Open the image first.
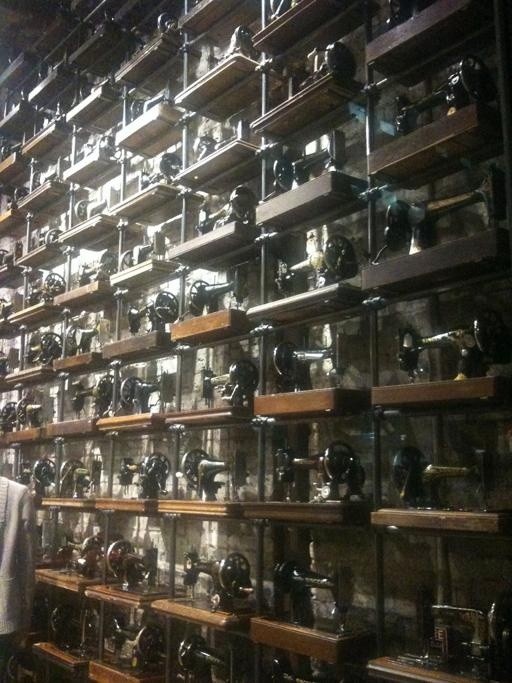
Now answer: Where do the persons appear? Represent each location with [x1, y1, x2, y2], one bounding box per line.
[0, 474, 38, 683]
